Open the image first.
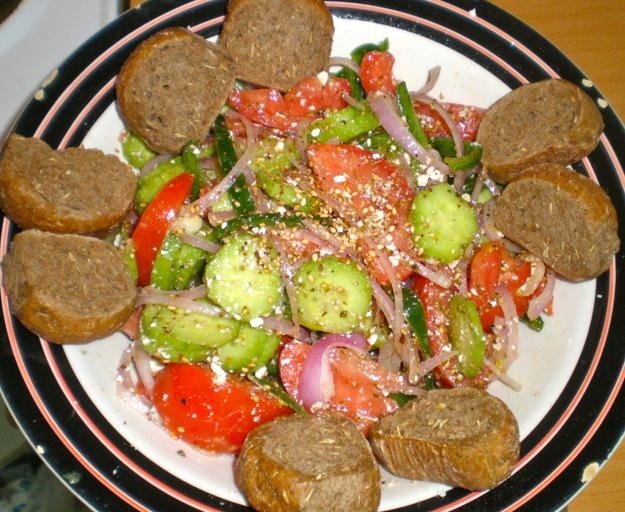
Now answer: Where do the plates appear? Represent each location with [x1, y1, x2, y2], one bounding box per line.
[1, 1, 625, 511]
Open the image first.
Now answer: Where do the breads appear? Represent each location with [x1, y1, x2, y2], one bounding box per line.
[231, 413, 382, 511]
[0, 133, 138, 234]
[474, 78, 603, 185]
[1, 229, 137, 345]
[493, 163, 620, 283]
[369, 389, 523, 492]
[111, 28, 240, 157]
[221, 3, 334, 87]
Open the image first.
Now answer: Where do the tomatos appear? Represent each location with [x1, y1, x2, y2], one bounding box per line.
[123, 51, 551, 455]
